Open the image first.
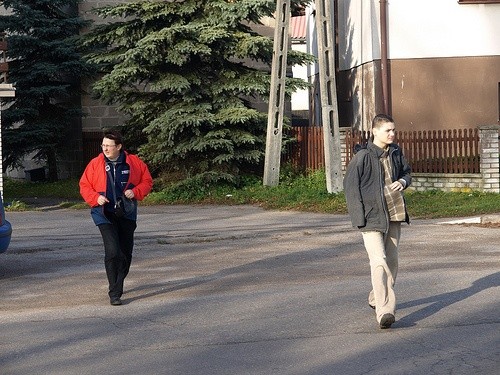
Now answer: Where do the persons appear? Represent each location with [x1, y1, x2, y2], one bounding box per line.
[80, 127, 152, 306]
[343, 113, 413, 329]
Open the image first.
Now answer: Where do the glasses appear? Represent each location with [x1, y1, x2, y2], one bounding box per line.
[100, 145, 115, 148]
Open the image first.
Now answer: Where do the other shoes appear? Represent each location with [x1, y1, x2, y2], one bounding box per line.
[111, 297, 122, 304]
[380, 313, 395, 329]
[369, 304, 375, 309]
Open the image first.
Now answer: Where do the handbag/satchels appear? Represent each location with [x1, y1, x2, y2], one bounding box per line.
[106, 163, 134, 217]
